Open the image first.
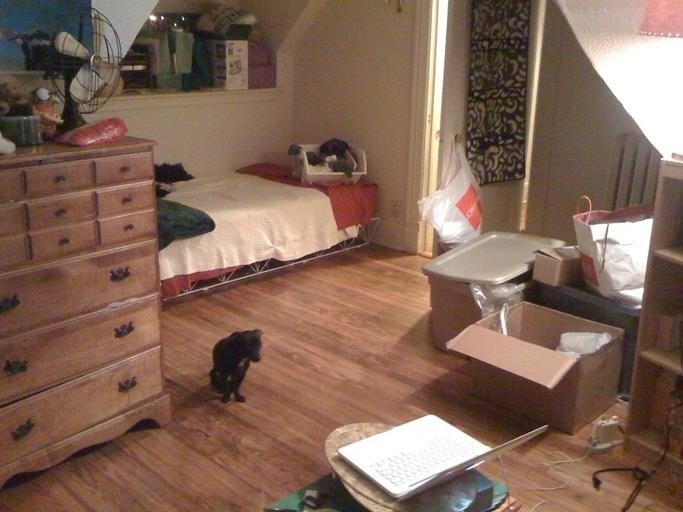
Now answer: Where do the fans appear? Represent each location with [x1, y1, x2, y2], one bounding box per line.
[40, 2, 124, 136]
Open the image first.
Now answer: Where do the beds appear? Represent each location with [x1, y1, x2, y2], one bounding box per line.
[152, 158, 383, 301]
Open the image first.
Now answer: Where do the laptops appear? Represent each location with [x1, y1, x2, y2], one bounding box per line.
[336, 414, 549, 501]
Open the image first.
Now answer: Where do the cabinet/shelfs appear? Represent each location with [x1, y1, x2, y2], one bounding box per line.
[0, 125, 174, 486]
[621, 149, 682, 471]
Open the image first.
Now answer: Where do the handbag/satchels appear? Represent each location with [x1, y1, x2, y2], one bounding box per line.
[572, 201, 654, 318]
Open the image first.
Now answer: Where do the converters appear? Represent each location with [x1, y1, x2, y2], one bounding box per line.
[598, 418, 618, 443]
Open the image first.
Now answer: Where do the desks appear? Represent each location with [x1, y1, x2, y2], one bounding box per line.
[259, 463, 525, 511]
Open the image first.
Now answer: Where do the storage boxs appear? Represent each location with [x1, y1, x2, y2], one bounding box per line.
[204, 37, 250, 93]
[290, 139, 367, 185]
[420, 229, 571, 357]
[529, 243, 581, 289]
[444, 298, 627, 437]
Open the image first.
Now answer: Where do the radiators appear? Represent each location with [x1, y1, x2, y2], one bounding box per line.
[605, 130, 662, 216]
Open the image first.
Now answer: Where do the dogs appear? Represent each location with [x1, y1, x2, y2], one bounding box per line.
[207, 328, 264, 402]
